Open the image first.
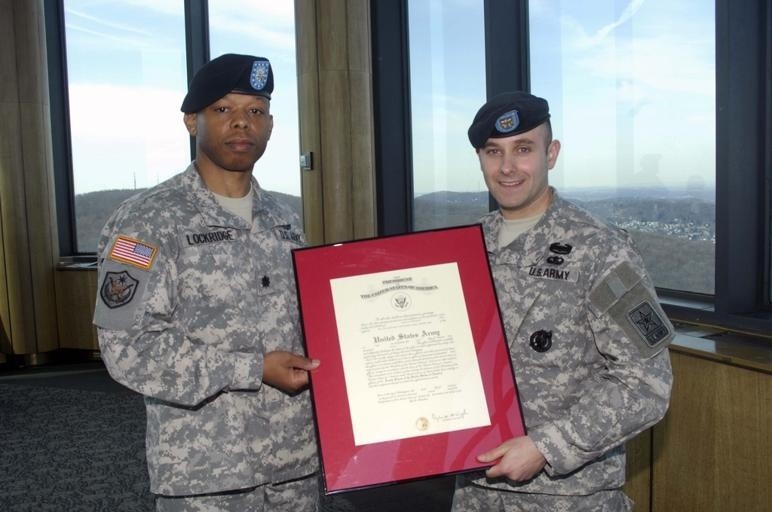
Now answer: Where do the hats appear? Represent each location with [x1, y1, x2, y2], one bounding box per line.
[181, 53, 274, 115]
[467, 92, 552, 148]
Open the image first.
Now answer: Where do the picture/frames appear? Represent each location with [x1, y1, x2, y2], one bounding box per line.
[290, 221, 528, 497]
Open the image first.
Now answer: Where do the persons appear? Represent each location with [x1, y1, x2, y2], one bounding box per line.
[92, 53, 321, 512]
[450, 92, 676, 512]
